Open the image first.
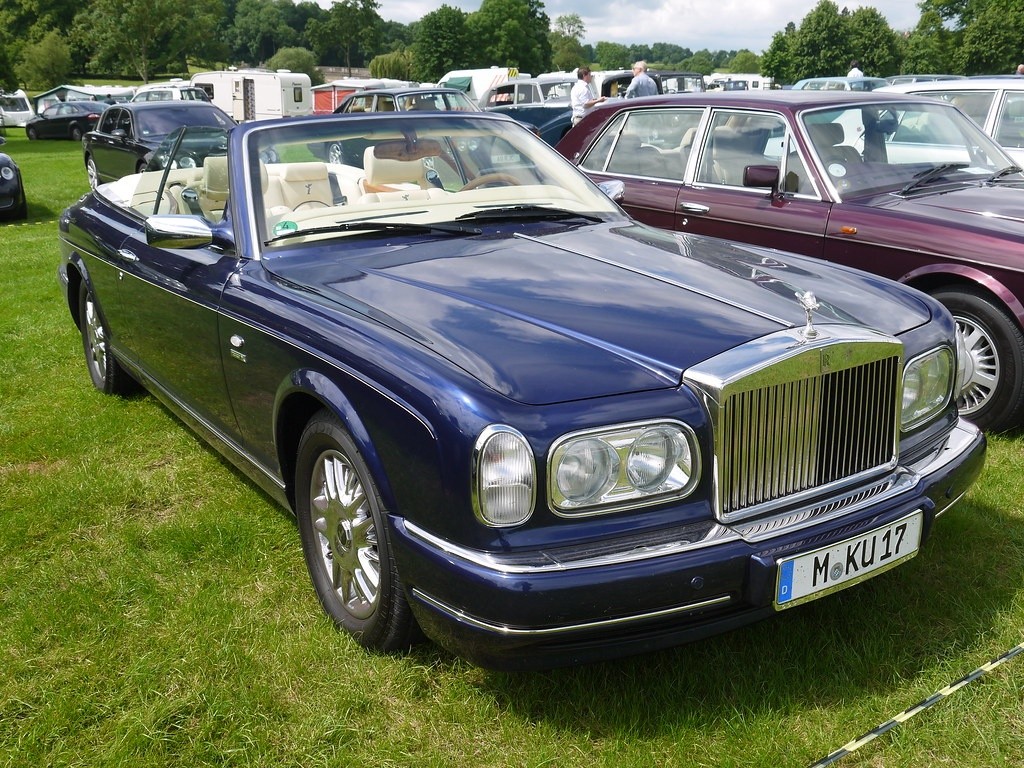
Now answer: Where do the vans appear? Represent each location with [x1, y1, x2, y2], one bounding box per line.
[191, 63, 1024, 172]
[128, 87, 211, 103]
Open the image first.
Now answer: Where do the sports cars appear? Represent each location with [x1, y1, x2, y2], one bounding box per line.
[58, 112, 987, 672]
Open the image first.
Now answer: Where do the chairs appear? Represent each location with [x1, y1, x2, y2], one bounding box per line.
[950, 95, 1023, 146]
[349, 99, 440, 115]
[594, 123, 863, 198]
[168, 147, 453, 225]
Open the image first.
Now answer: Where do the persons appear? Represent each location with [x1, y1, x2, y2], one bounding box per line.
[844, 61, 864, 90]
[1015, 64, 1024, 75]
[624, 61, 658, 144]
[570, 67, 607, 128]
[104, 94, 116, 105]
[90, 96, 95, 101]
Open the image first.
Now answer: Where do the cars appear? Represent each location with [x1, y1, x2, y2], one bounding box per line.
[84, 101, 238, 191]
[26, 101, 111, 141]
[554, 90, 1024, 433]
